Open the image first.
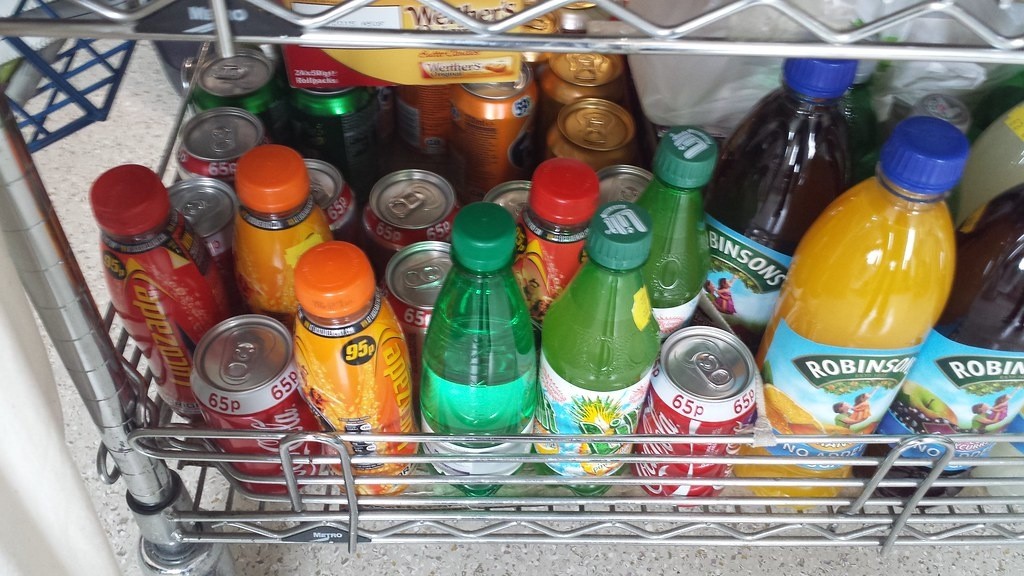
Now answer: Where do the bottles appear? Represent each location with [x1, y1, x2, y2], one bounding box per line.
[419, 201, 537, 503]
[699, 40, 883, 365]
[629, 125, 718, 345]
[534, 202, 660, 497]
[513, 157, 613, 354]
[288, 239, 421, 503]
[727, 92, 1023, 517]
[89, 164, 235, 440]
[229, 142, 337, 327]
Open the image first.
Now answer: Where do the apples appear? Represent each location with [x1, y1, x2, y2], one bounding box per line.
[901, 382, 957, 425]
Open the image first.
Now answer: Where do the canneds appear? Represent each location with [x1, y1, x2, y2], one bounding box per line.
[164, 0, 761, 498]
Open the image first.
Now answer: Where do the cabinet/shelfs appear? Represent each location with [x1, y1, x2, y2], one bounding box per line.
[0, 0, 1024, 576]
